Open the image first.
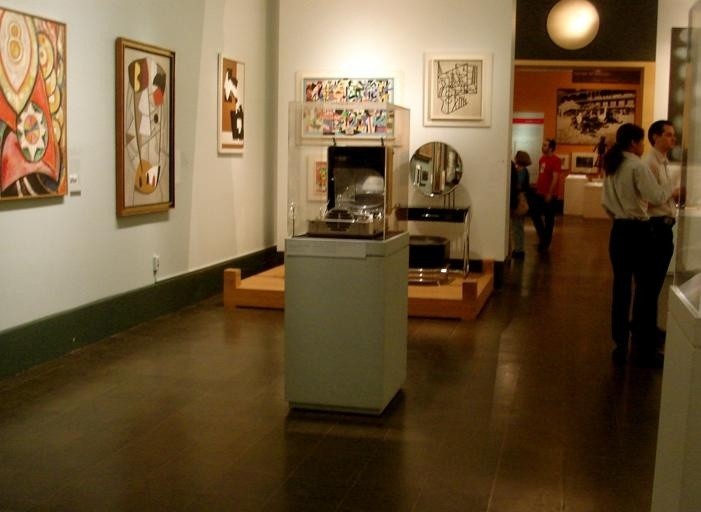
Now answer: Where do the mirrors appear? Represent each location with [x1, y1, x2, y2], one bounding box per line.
[408, 141, 463, 208]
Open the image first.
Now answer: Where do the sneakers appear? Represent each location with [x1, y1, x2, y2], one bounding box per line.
[612, 328, 665, 366]
[536, 242, 551, 252]
[512, 249, 525, 257]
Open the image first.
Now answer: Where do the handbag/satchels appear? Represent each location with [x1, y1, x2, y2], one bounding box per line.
[514, 192, 530, 216]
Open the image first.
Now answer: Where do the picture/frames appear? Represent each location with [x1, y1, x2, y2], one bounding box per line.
[116, 37, 176, 217]
[423, 49, 493, 128]
[0, 6, 69, 200]
[571, 151, 598, 174]
[217, 51, 246, 154]
[296, 71, 395, 139]
[307, 151, 329, 203]
[410, 142, 462, 198]
[572, 68, 641, 84]
[556, 155, 569, 170]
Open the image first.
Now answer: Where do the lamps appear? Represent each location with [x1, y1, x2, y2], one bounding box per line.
[546, 0, 600, 51]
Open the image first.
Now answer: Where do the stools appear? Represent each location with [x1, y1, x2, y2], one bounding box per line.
[408, 235, 451, 286]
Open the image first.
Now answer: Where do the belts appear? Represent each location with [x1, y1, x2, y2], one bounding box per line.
[654, 215, 675, 226]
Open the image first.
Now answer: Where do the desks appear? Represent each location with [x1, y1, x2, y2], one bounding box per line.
[408, 205, 471, 279]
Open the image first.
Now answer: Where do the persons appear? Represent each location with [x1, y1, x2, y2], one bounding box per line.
[512, 150, 533, 258]
[638, 120, 682, 345]
[535, 139, 562, 251]
[600, 124, 678, 370]
